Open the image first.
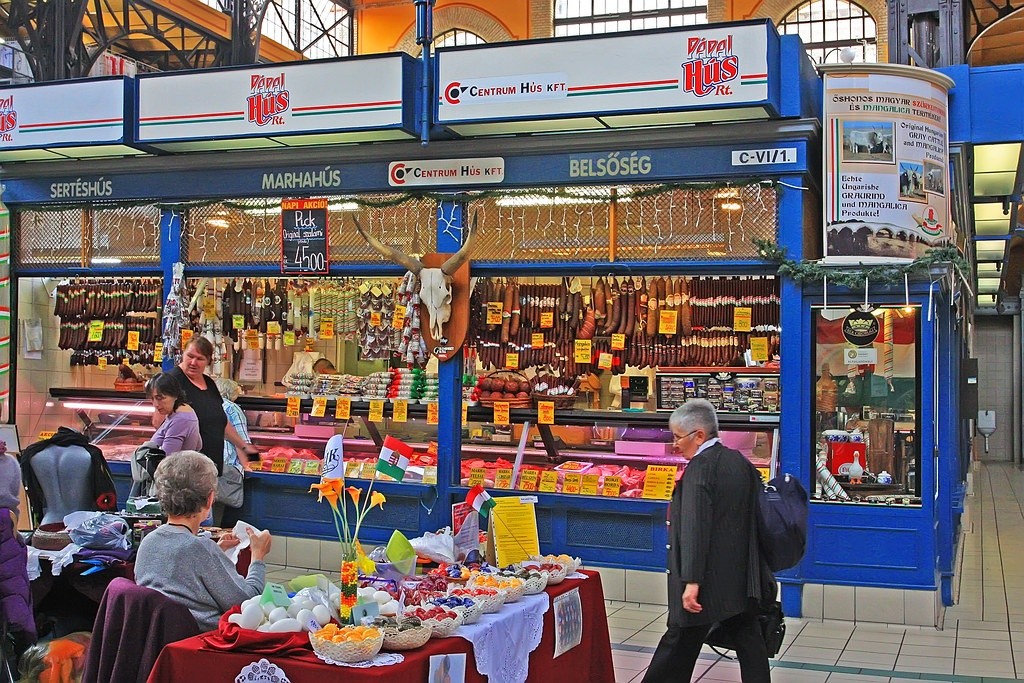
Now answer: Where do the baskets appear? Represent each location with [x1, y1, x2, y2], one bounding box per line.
[361, 614, 432, 650]
[421, 555, 581, 636]
[530, 391, 577, 409]
[475, 369, 533, 408]
[309, 624, 385, 662]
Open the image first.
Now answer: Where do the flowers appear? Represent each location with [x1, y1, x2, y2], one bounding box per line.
[308, 477, 386, 561]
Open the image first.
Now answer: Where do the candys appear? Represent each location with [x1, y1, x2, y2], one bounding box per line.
[314, 623, 380, 654]
[379, 562, 521, 621]
[524, 554, 572, 578]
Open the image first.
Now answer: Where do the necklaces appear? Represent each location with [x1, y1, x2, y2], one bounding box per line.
[167, 523, 192, 534]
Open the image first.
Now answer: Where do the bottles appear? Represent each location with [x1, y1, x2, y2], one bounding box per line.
[849, 451, 863, 479]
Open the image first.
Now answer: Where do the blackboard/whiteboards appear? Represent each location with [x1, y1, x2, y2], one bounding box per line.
[281, 208, 328, 274]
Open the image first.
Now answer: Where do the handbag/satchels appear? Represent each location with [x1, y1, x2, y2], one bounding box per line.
[758, 474, 807, 569]
[703, 600, 786, 658]
[216, 466, 243, 508]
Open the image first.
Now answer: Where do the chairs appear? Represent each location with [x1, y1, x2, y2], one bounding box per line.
[79, 576, 201, 683]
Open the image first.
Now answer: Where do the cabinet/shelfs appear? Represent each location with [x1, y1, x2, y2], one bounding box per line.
[654, 367, 780, 415]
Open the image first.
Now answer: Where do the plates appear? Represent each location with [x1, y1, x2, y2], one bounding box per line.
[553, 461, 593, 473]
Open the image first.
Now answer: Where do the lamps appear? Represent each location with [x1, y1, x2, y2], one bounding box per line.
[807, 46, 856, 64]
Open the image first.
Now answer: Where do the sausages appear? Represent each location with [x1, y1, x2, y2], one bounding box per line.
[54, 280, 163, 384]
[531, 374, 581, 397]
[468, 277, 780, 379]
[189, 272, 430, 377]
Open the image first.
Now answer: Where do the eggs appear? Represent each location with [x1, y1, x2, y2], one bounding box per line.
[228, 594, 330, 632]
[331, 587, 399, 614]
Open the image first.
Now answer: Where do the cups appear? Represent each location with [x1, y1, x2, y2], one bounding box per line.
[849, 432, 861, 443]
[877, 471, 891, 484]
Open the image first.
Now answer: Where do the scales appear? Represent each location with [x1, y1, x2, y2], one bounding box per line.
[615, 376, 676, 457]
[531, 435, 566, 450]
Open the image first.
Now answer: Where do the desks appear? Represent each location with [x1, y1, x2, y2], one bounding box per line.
[21, 518, 251, 637]
[147, 547, 616, 683]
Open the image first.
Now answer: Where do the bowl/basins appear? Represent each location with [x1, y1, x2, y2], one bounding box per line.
[359, 555, 418, 581]
[595, 422, 628, 440]
[822, 432, 848, 443]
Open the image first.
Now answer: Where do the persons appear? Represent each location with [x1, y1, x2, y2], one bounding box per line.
[640, 398, 770, 683]
[312, 359, 343, 375]
[170, 335, 258, 527]
[145, 371, 202, 460]
[213, 378, 252, 528]
[133, 452, 273, 633]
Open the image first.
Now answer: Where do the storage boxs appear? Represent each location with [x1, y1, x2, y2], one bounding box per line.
[295, 424, 360, 439]
[358, 553, 419, 592]
[615, 441, 675, 456]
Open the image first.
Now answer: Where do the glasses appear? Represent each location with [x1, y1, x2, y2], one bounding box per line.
[673, 430, 697, 441]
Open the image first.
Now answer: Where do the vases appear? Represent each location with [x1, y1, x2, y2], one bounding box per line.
[338, 553, 359, 626]
